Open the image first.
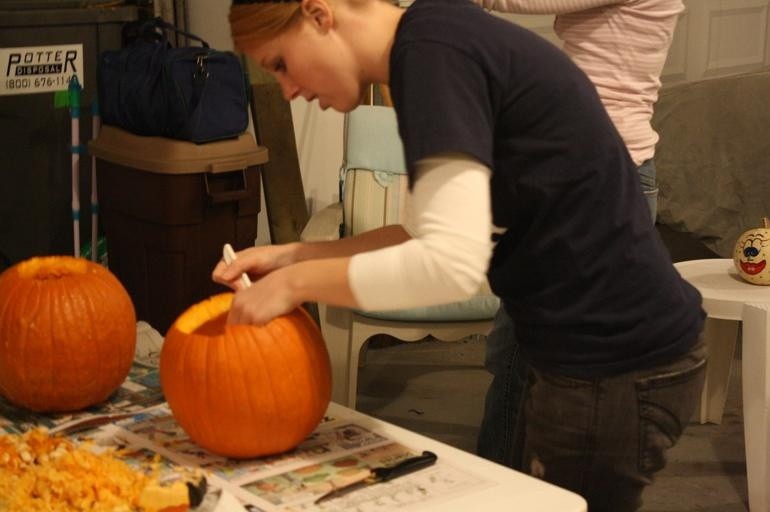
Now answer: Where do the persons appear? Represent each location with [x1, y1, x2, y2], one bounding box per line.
[467, 0, 686, 473]
[210, 0, 711, 511]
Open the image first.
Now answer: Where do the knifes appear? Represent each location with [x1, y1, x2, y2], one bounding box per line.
[311, 451, 438, 505]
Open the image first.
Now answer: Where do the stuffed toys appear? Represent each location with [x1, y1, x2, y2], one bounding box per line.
[733, 227, 770, 286]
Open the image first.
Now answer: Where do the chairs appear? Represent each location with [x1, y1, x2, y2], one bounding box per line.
[300, 105, 501, 410]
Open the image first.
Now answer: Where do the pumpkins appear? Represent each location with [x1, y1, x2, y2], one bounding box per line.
[160, 290, 333, 457]
[0, 255, 136, 413]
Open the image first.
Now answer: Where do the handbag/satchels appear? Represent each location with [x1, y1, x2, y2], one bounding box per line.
[96, 16, 249, 144]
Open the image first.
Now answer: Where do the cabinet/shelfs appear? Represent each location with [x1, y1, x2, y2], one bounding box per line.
[0, 0, 140, 272]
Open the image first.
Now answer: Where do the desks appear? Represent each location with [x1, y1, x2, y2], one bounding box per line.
[672, 257, 770, 512]
[0, 355, 588, 512]
